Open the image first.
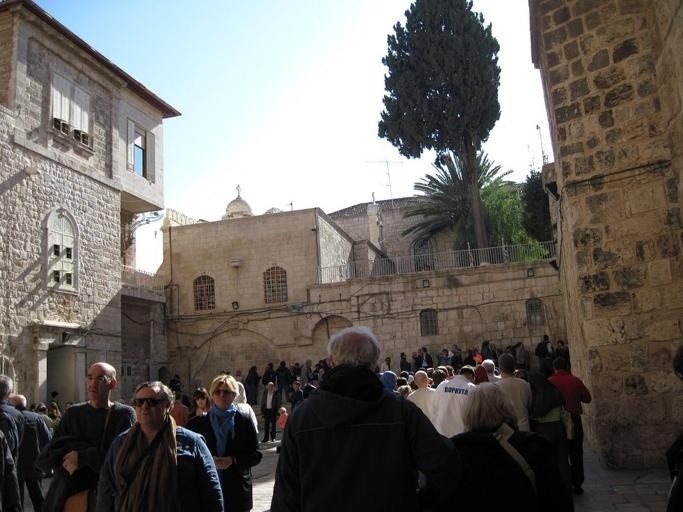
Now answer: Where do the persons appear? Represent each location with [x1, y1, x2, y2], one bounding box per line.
[664, 345, 683, 512]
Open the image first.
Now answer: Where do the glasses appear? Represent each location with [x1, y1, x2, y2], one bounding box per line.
[214, 390, 230, 395]
[87, 377, 104, 381]
[133, 399, 169, 407]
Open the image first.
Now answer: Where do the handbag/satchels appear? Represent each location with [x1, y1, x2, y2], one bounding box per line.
[63, 489, 88, 512]
[561, 405, 575, 440]
